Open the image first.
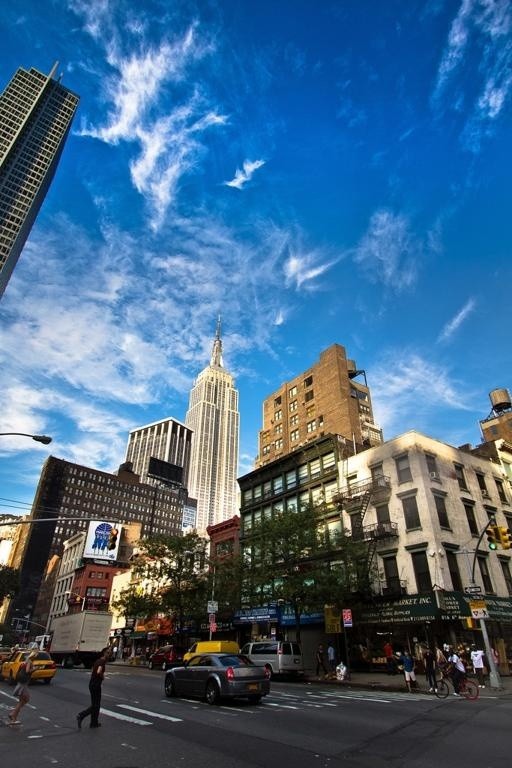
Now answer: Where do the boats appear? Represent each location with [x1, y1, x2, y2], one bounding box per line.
[485, 527, 498, 550]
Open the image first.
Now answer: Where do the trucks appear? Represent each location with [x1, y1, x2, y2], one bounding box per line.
[46, 610, 114, 669]
[30, 635, 51, 651]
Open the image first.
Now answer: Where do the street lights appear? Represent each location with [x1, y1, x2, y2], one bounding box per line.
[184, 549, 216, 641]
[65, 589, 86, 611]
[0, 431, 53, 445]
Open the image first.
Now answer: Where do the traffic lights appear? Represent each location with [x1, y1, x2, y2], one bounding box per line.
[108, 528, 119, 550]
[11, 619, 15, 626]
[498, 526, 512, 550]
[461, 619, 471, 628]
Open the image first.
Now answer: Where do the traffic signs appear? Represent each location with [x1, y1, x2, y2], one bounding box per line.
[463, 585, 483, 594]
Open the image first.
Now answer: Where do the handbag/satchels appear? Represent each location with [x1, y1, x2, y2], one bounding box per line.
[15, 668, 29, 684]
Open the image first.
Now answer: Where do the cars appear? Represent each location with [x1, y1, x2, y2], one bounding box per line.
[146, 638, 305, 707]
[0, 645, 57, 684]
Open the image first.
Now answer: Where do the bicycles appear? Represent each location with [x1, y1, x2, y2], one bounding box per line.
[430, 664, 482, 700]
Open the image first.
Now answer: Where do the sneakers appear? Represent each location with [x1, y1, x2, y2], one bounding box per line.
[7, 714, 24, 726]
[75, 715, 102, 730]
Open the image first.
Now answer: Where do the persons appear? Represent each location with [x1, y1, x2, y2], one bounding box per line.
[77, 647, 110, 731]
[113, 646, 151, 662]
[316, 642, 499, 696]
[8, 648, 40, 725]
